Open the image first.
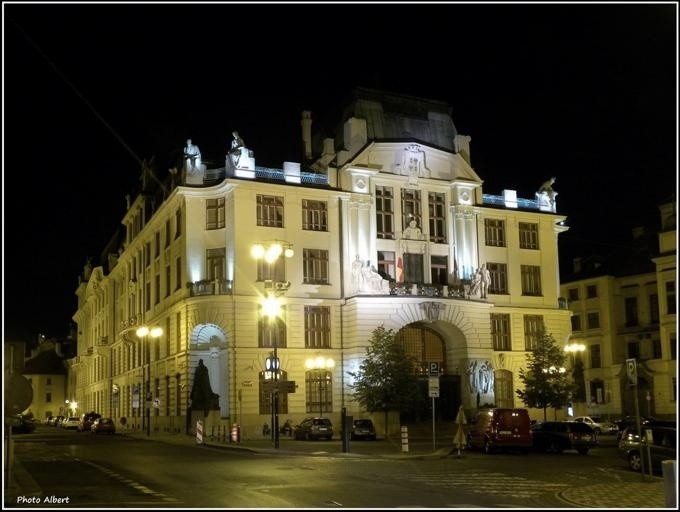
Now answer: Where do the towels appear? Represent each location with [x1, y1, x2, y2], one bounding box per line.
[662, 460, 676, 507]
[229, 426, 240, 441]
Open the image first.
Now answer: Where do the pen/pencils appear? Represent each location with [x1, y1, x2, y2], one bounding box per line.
[468, 408, 533, 454]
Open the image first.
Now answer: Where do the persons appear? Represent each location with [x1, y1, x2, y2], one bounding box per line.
[282, 418, 294, 438]
[190, 358, 219, 413]
[470, 268, 482, 296]
[464, 360, 495, 395]
[262, 422, 271, 437]
[477, 262, 491, 300]
[360, 259, 381, 291]
[183, 138, 202, 177]
[82, 260, 93, 281]
[402, 220, 422, 240]
[54, 416, 62, 432]
[227, 130, 255, 169]
[539, 176, 558, 208]
[351, 253, 364, 293]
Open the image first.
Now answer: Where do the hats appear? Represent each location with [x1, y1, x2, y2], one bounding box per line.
[45, 413, 116, 434]
[17, 415, 35, 432]
[294, 416, 334, 441]
[531, 415, 676, 471]
[351, 419, 376, 440]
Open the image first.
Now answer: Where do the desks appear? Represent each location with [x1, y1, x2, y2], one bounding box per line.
[540, 365, 565, 421]
[263, 351, 282, 443]
[255, 237, 295, 449]
[136, 324, 162, 437]
[563, 342, 586, 415]
